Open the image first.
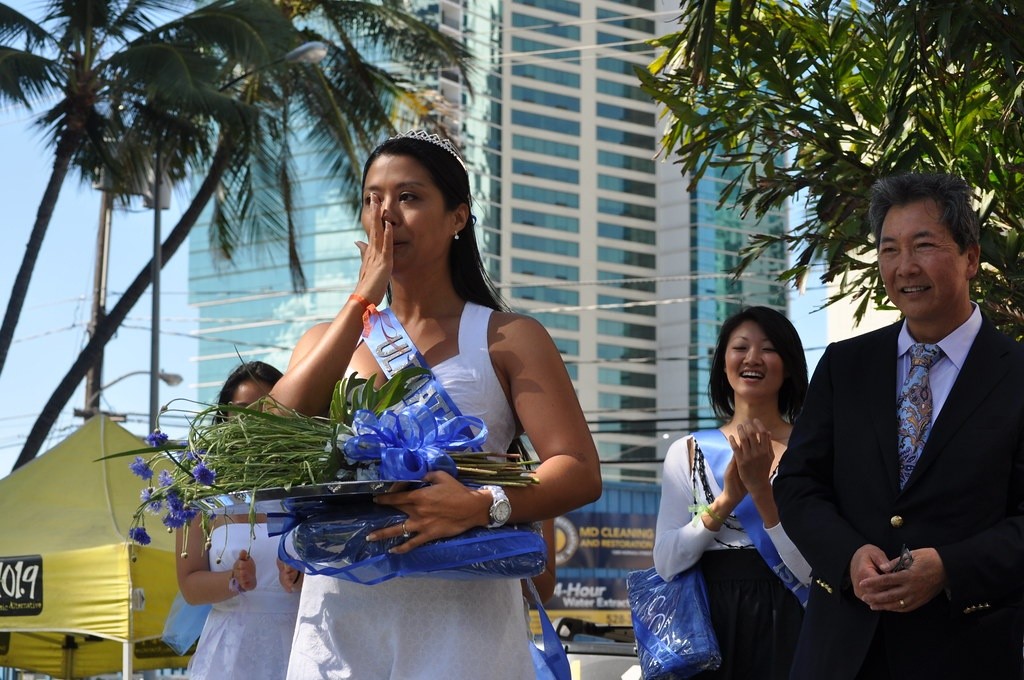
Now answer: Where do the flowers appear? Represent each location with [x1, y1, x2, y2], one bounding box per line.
[890, 516, 913, 573]
[91, 343, 544, 566]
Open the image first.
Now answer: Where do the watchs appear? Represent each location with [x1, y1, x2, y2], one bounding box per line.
[478, 484, 512, 529]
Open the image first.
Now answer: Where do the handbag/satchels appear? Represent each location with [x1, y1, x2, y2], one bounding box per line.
[161, 590, 213, 657]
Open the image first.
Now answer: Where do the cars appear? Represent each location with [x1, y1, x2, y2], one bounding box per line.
[535, 616, 641, 680]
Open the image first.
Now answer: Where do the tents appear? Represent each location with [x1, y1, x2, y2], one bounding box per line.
[0, 412, 199, 680]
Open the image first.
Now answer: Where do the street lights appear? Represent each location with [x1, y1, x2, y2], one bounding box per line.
[83, 371, 184, 424]
[145, 39, 331, 450]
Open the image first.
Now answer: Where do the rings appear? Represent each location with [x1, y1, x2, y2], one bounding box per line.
[897, 599, 907, 608]
[402, 523, 411, 537]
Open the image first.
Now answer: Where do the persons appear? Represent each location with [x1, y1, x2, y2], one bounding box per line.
[652, 175, 1024, 679]
[175, 128, 602, 680]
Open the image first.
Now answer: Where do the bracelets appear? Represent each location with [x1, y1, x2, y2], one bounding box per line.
[346, 292, 407, 354]
[688, 490, 726, 528]
[227, 570, 247, 599]
[293, 570, 300, 584]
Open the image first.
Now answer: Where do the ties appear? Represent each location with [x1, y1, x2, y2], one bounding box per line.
[897, 342, 947, 488]
